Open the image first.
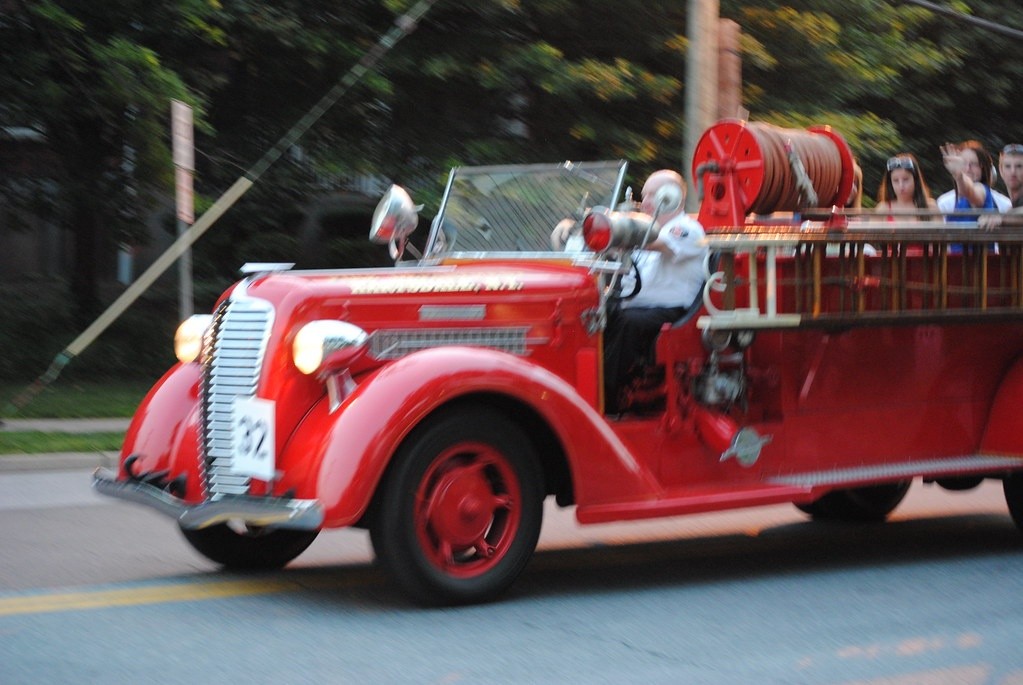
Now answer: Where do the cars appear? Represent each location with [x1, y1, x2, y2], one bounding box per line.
[93, 117, 1023, 603]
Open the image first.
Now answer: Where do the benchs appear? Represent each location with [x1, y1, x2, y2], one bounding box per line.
[652, 250, 736, 391]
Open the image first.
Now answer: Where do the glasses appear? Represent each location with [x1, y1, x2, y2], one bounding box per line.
[886, 157, 914, 173]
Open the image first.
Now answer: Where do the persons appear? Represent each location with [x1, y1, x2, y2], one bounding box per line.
[549, 168, 710, 418]
[795, 138, 1022, 254]
